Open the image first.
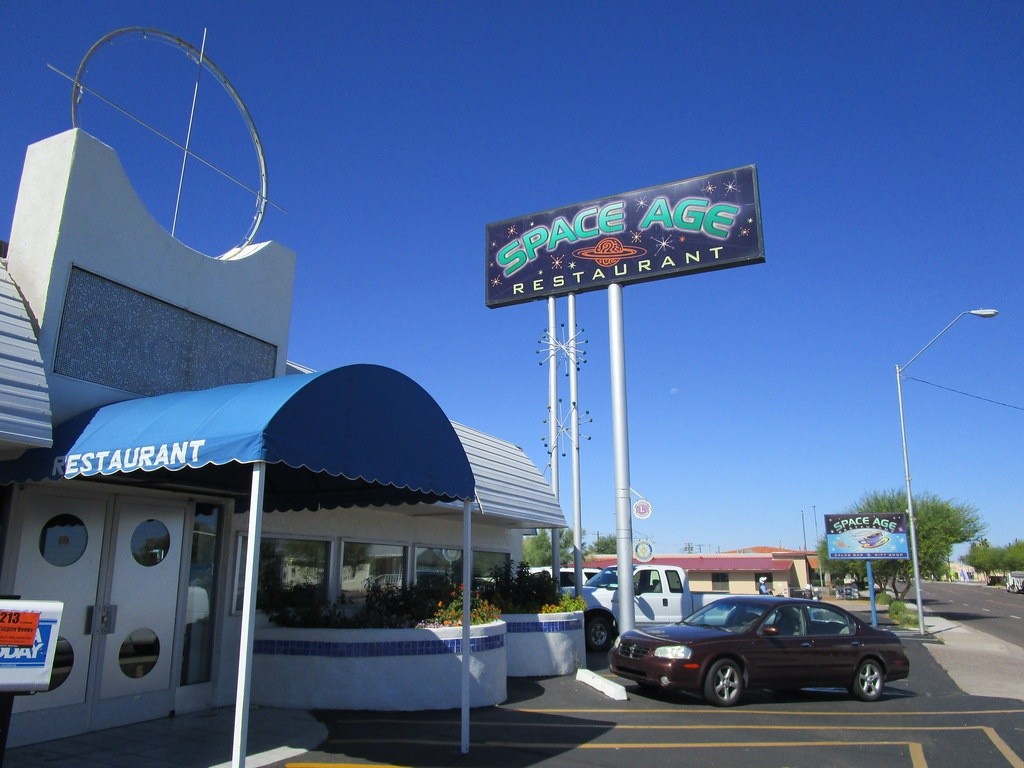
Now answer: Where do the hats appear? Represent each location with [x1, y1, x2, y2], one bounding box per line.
[760, 577, 767, 584]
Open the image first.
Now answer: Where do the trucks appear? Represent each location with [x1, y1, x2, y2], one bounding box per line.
[1006, 571, 1024, 593]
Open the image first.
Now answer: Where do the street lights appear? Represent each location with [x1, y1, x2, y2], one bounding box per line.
[895, 308, 999, 636]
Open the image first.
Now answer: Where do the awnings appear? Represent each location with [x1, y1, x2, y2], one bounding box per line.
[0, 363, 476, 768]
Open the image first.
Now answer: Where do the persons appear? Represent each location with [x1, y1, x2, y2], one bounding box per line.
[759, 577, 771, 595]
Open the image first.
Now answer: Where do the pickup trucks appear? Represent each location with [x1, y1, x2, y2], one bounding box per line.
[567, 564, 736, 651]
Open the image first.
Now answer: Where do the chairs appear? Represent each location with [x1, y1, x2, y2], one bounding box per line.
[776, 607, 800, 636]
[738, 612, 760, 627]
[649, 580, 662, 593]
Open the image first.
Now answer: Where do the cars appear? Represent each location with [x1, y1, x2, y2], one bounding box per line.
[607, 595, 910, 707]
[559, 568, 602, 598]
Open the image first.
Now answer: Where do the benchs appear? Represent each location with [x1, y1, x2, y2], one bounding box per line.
[810, 620, 846, 634]
